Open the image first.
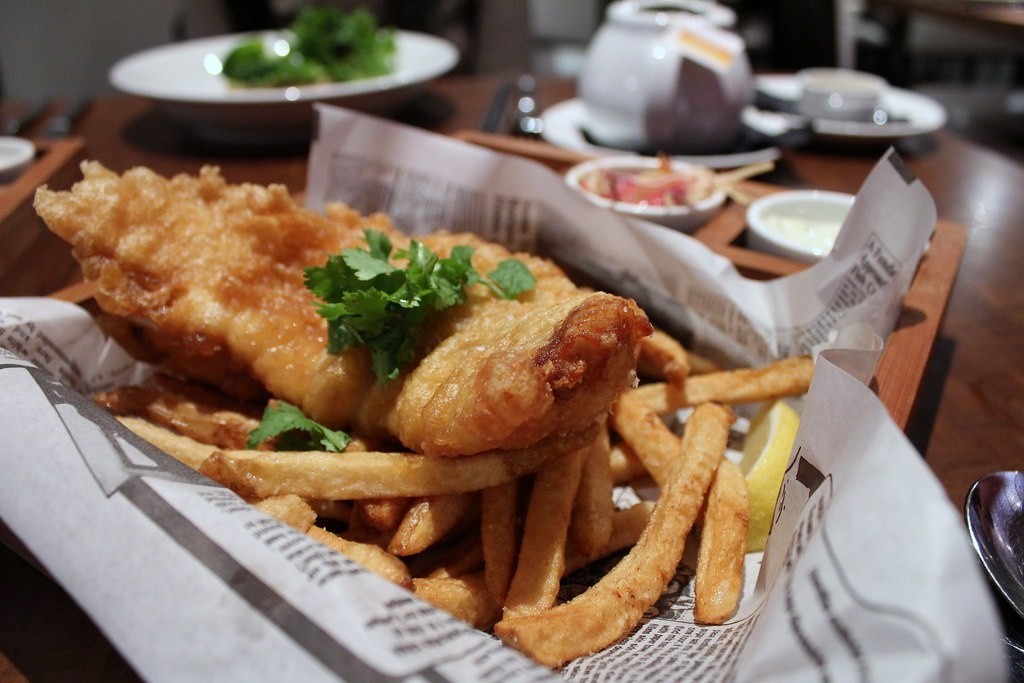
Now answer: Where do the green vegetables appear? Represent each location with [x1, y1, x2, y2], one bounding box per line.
[225, 10, 391, 87]
[245, 236, 534, 450]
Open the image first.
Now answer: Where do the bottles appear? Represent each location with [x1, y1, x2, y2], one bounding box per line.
[576, 0, 748, 149]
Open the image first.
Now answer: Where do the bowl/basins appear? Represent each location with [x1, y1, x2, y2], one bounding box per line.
[746, 189, 855, 259]
[565, 158, 729, 229]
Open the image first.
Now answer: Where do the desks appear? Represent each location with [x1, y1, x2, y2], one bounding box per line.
[0, 81, 1024, 683]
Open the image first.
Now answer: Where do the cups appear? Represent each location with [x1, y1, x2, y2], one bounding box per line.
[794, 70, 886, 120]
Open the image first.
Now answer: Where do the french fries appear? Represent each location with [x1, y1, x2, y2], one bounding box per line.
[127, 333, 815, 664]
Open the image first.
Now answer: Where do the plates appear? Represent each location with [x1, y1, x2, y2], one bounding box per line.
[539, 97, 779, 167]
[740, 73, 948, 137]
[108, 35, 460, 106]
[965, 471, 1024, 617]
[0, 136, 35, 179]
